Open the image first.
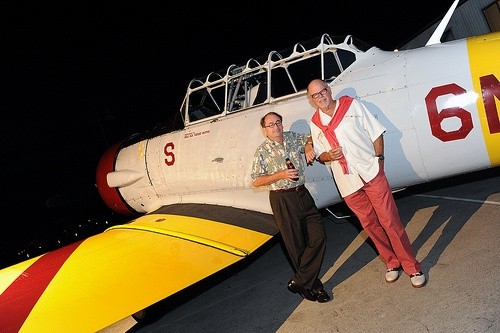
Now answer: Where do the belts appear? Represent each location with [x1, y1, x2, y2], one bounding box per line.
[270, 184, 305, 193]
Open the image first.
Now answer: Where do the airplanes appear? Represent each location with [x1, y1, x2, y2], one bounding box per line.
[0, 1, 500, 333]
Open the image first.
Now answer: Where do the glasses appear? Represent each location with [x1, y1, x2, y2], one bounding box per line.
[311, 87, 327, 99]
[265, 121, 281, 128]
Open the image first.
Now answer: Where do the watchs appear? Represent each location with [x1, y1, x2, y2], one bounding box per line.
[376, 154, 384, 159]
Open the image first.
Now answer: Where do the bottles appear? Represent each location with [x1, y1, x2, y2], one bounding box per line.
[286, 158, 299, 181]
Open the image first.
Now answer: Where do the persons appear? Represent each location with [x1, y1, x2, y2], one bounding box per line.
[307, 79, 426, 288]
[251, 112, 330, 302]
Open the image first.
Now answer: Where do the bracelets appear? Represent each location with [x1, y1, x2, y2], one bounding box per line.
[306, 141, 313, 147]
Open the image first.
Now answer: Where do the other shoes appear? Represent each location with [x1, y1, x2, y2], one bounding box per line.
[385, 267, 398, 283]
[410, 271, 426, 288]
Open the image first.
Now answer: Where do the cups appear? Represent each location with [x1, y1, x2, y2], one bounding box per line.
[331, 142, 343, 158]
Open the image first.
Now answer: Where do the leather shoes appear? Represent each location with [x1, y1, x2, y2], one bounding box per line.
[287, 279, 317, 301]
[311, 289, 330, 303]
